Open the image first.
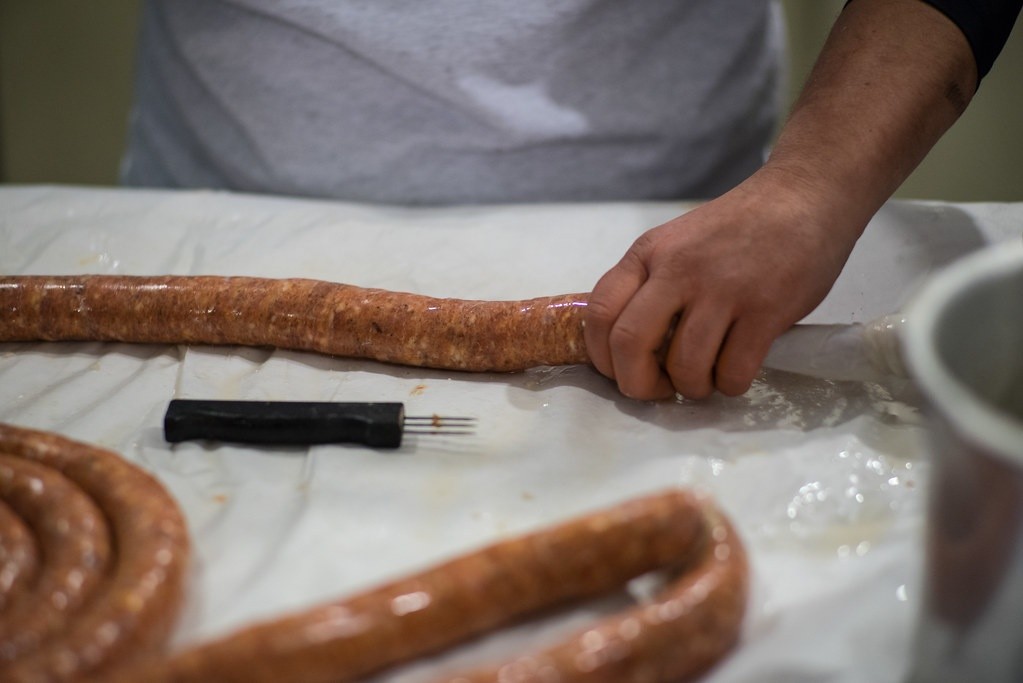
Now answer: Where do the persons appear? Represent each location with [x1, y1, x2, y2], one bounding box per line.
[123, 0, 1022, 398]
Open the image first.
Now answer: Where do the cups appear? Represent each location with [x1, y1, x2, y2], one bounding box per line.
[903, 238, 1023, 683]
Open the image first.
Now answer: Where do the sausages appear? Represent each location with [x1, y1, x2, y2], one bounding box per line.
[0, 422, 750, 683]
[0, 273, 674, 370]
